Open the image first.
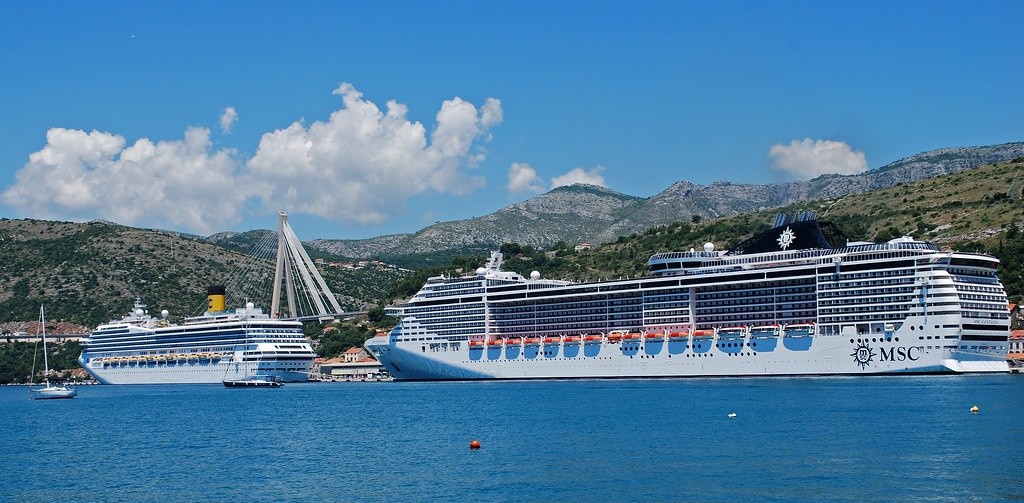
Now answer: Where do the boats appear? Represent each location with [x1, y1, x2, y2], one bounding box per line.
[222, 380, 284, 387]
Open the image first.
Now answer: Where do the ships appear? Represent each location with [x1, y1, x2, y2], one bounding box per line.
[363, 235, 1011, 381]
[76, 320, 316, 383]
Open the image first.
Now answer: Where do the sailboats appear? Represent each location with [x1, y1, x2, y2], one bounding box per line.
[27, 304, 78, 400]
